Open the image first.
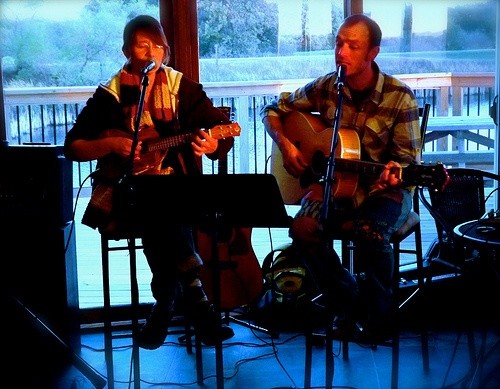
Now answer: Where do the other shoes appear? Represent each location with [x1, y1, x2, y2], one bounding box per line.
[183, 304, 234, 347]
[138, 304, 175, 349]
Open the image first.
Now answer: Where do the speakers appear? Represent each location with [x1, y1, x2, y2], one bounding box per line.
[0, 144, 79, 388]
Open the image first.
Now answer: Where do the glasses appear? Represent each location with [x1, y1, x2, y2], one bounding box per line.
[134, 41, 164, 52]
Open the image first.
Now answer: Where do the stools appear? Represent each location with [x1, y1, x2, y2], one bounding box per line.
[341, 103, 431, 356]
[102, 229, 194, 337]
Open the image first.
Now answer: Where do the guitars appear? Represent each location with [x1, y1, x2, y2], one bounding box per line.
[195, 107, 264, 310]
[99, 120, 241, 186]
[271, 111, 449, 206]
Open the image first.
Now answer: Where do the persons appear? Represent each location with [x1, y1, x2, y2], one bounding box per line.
[259, 14, 424, 333]
[63, 14, 237, 350]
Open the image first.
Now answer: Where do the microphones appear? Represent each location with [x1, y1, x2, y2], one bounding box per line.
[335, 62, 348, 88]
[141, 59, 155, 74]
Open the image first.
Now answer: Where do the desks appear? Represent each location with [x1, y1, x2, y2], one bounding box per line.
[454, 221, 500, 337]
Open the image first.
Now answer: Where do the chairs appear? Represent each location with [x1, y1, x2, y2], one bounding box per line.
[418, 168, 500, 337]
